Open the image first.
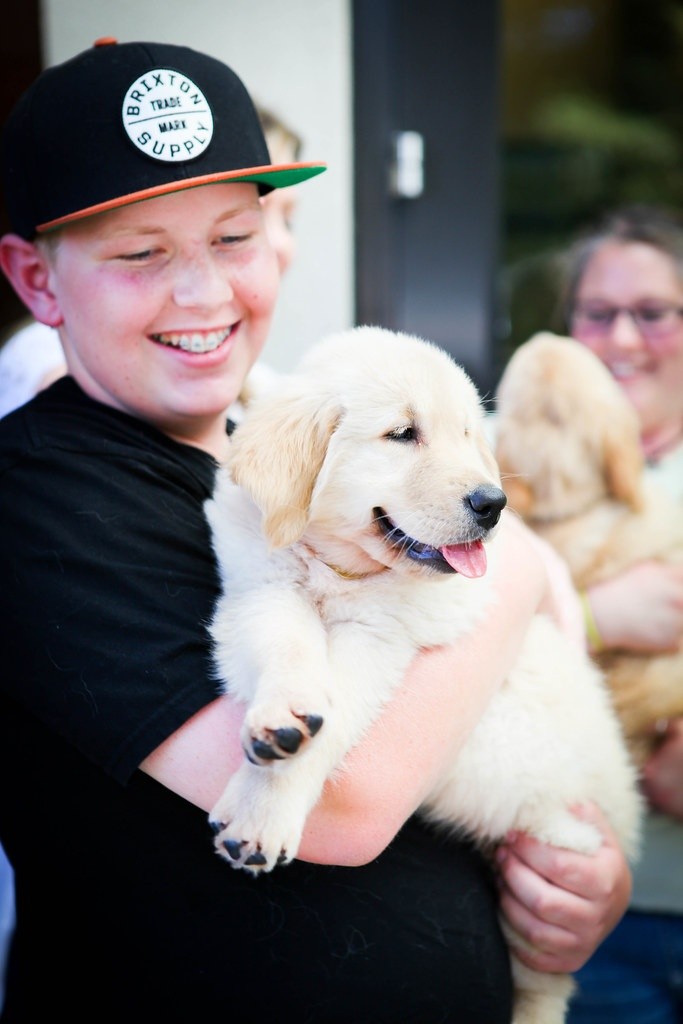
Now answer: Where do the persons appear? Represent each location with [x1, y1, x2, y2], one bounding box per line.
[482, 221, 682, 1023]
[0, 104, 305, 420]
[0, 43, 631, 1024]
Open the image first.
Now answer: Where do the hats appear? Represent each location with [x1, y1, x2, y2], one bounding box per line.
[0, 34, 332, 232]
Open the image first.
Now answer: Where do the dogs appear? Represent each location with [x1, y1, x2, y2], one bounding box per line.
[205, 324, 683, 1024]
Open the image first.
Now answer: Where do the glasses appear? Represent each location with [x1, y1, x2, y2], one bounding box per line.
[569, 302, 682, 338]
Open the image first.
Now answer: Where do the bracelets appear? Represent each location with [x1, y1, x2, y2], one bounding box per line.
[578, 589, 604, 658]
